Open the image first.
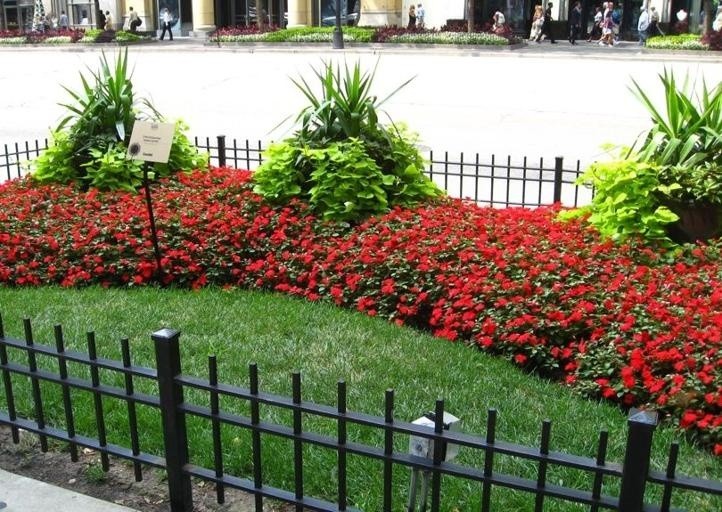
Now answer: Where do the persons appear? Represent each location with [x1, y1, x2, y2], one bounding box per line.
[492, 7, 506, 30]
[105, 10, 113, 31]
[128, 7, 138, 32]
[35, 10, 70, 35]
[416, 3, 425, 29]
[406, 5, 418, 29]
[526, 0, 722, 47]
[75, 4, 90, 25]
[158, 8, 173, 42]
[100, 10, 106, 30]
